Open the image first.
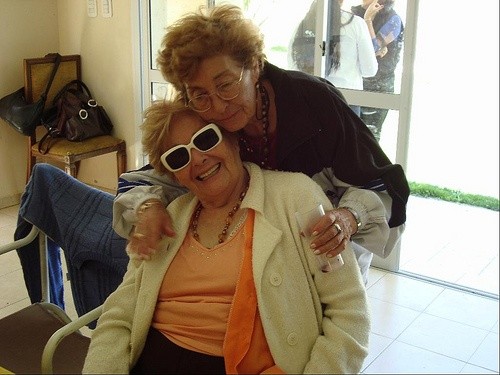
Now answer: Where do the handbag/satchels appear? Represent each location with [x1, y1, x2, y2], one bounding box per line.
[38, 80, 113, 155]
[0, 53, 61, 136]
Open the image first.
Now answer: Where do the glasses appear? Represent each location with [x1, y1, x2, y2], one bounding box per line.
[184, 66, 244, 112]
[160, 122, 223, 172]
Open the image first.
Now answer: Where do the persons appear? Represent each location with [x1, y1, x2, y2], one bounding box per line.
[292, 0, 405, 146]
[157, 4, 410, 291]
[80, 92, 373, 374]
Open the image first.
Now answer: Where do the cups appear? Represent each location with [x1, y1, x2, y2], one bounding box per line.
[295, 202, 344, 273]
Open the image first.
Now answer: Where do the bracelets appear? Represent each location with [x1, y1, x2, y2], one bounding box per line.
[339, 206, 362, 239]
[137, 199, 160, 216]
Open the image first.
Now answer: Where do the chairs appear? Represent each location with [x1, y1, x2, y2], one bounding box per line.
[23, 54, 128, 183]
[0, 164, 129, 375]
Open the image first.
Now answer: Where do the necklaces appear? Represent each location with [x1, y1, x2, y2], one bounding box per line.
[191, 182, 249, 251]
[232, 80, 270, 170]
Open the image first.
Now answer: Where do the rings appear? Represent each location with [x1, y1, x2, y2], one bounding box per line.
[131, 233, 145, 241]
[333, 222, 340, 231]
